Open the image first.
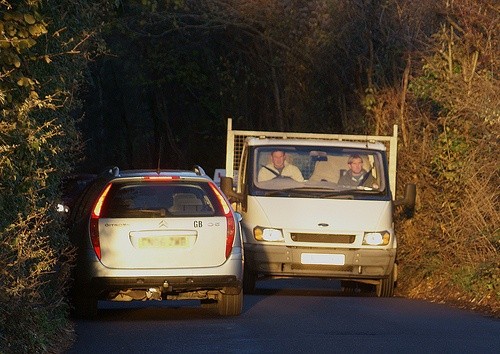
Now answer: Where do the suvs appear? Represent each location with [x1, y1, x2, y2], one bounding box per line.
[56, 163, 246, 316]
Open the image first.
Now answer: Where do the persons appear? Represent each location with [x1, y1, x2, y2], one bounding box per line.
[257, 150, 304, 183]
[337, 154, 379, 188]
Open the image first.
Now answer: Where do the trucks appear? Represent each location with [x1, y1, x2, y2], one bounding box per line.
[214, 118, 417, 299]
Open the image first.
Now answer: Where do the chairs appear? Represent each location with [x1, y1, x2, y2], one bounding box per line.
[258, 150, 293, 168]
[171, 192, 198, 214]
[315, 155, 374, 184]
